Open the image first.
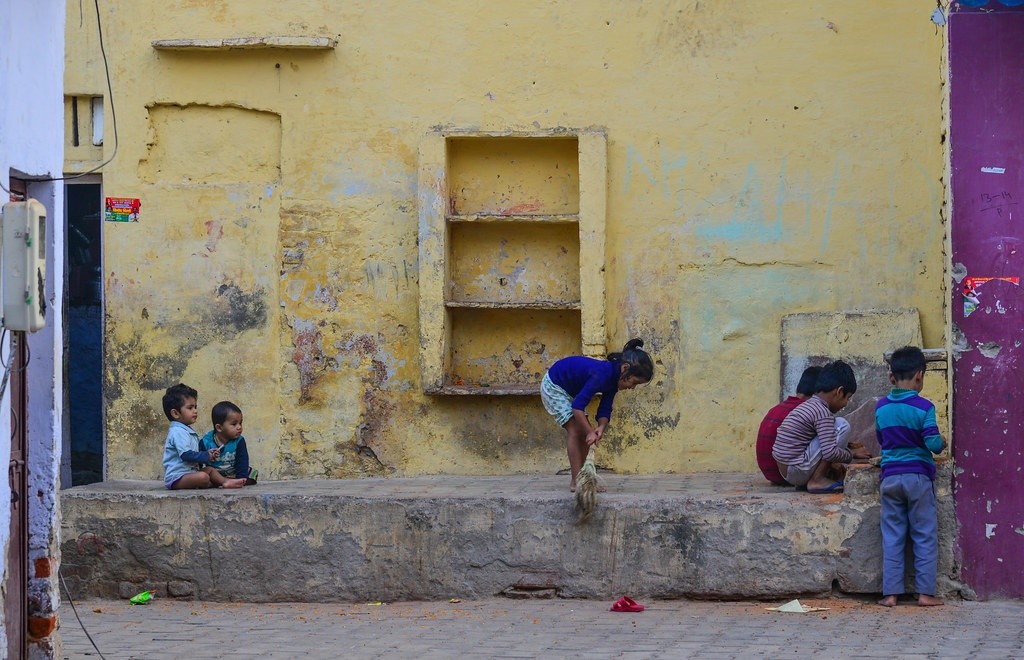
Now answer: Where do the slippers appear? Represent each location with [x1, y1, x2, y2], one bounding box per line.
[611, 597, 645, 612]
[809, 481, 844, 493]
[796, 485, 807, 492]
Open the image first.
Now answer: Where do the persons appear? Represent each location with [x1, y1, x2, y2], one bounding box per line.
[161, 384, 247, 489]
[875, 346, 946, 607]
[198, 402, 249, 479]
[757, 366, 823, 485]
[772, 360, 871, 492]
[541, 338, 653, 492]
